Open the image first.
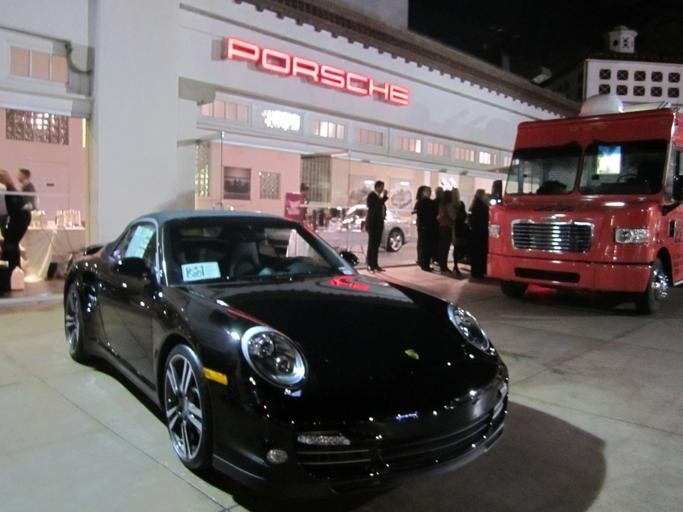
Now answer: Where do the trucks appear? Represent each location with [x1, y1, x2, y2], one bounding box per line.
[479, 91, 682, 317]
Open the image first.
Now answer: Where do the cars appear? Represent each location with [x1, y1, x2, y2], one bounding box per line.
[313, 198, 413, 256]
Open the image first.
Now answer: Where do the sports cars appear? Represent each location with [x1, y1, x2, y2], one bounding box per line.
[59, 205, 515, 502]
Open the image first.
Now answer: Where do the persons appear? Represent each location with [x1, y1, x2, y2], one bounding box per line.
[300, 182, 309, 216]
[0, 168, 37, 286]
[364, 180, 389, 270]
[411, 185, 492, 281]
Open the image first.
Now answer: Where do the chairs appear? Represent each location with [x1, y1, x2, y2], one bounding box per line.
[227, 236, 260, 276]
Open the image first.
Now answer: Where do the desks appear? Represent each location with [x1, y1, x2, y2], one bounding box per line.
[19, 223, 85, 283]
[285, 228, 368, 263]
